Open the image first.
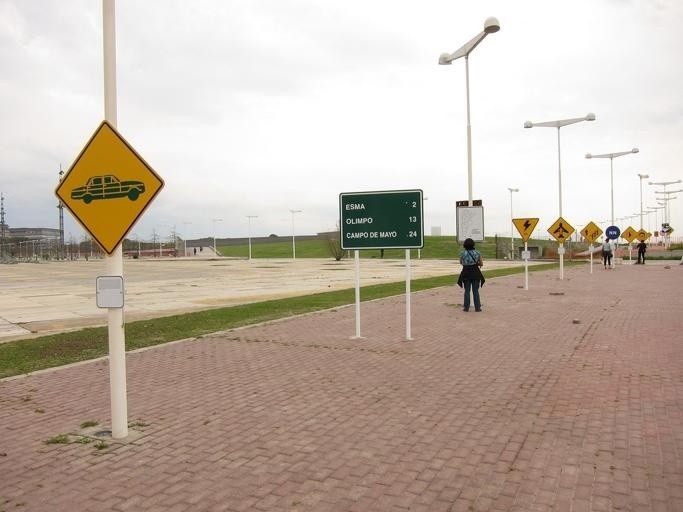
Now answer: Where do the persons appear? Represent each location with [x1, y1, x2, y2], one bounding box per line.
[601, 237, 613, 269]
[459, 238, 484, 312]
[636, 240, 647, 264]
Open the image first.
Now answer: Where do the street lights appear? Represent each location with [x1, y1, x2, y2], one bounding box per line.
[288, 208, 303, 260]
[523, 113, 596, 280]
[576, 179, 683, 250]
[507, 187, 519, 260]
[584, 148, 639, 268]
[208, 218, 223, 257]
[637, 174, 650, 264]
[244, 214, 258, 262]
[417, 197, 428, 260]
[0, 223, 189, 261]
[438, 17, 501, 246]
